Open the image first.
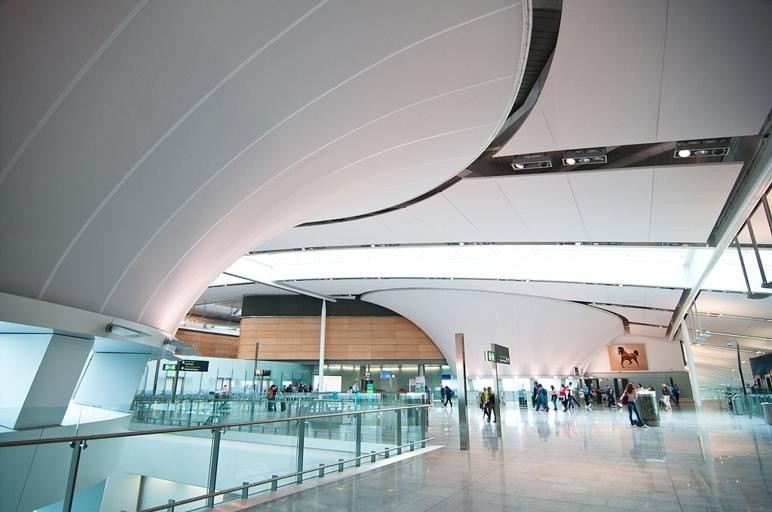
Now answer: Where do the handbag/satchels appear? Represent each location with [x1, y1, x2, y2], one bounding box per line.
[479, 401, 491, 409]
[579, 391, 584, 398]
[662, 390, 671, 395]
[621, 395, 628, 405]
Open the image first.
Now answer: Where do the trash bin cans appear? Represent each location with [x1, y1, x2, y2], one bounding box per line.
[636, 390, 660, 427]
[732, 394, 745, 415]
[761, 402, 772, 425]
[407, 392, 429, 428]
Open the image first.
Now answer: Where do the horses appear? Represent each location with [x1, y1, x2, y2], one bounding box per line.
[617, 346, 640, 368]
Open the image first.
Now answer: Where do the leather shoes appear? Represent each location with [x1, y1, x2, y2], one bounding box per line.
[482, 415, 496, 423]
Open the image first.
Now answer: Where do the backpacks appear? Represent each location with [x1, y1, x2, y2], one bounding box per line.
[450, 390, 454, 395]
[268, 389, 274, 399]
[543, 389, 547, 395]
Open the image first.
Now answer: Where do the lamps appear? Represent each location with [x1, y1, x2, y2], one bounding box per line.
[510, 141, 732, 172]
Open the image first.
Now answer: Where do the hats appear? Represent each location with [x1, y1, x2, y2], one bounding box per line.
[661, 384, 667, 387]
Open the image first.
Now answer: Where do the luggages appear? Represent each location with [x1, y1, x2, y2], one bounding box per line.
[617, 403, 622, 408]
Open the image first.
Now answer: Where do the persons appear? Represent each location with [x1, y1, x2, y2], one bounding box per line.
[284, 383, 313, 392]
[224, 385, 228, 391]
[638, 383, 643, 389]
[268, 385, 276, 410]
[530, 381, 604, 412]
[352, 382, 359, 391]
[648, 384, 654, 391]
[274, 386, 278, 410]
[533, 408, 572, 442]
[631, 428, 647, 472]
[425, 385, 431, 404]
[443, 385, 453, 407]
[483, 419, 489, 450]
[662, 383, 672, 409]
[623, 382, 650, 427]
[485, 386, 496, 422]
[481, 386, 488, 402]
[666, 383, 677, 407]
[674, 384, 680, 403]
[605, 384, 615, 406]
[487, 422, 498, 458]
[482, 405, 489, 418]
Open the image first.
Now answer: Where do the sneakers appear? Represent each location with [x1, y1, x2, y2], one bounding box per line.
[631, 424, 649, 428]
[563, 406, 574, 412]
[535, 407, 557, 412]
